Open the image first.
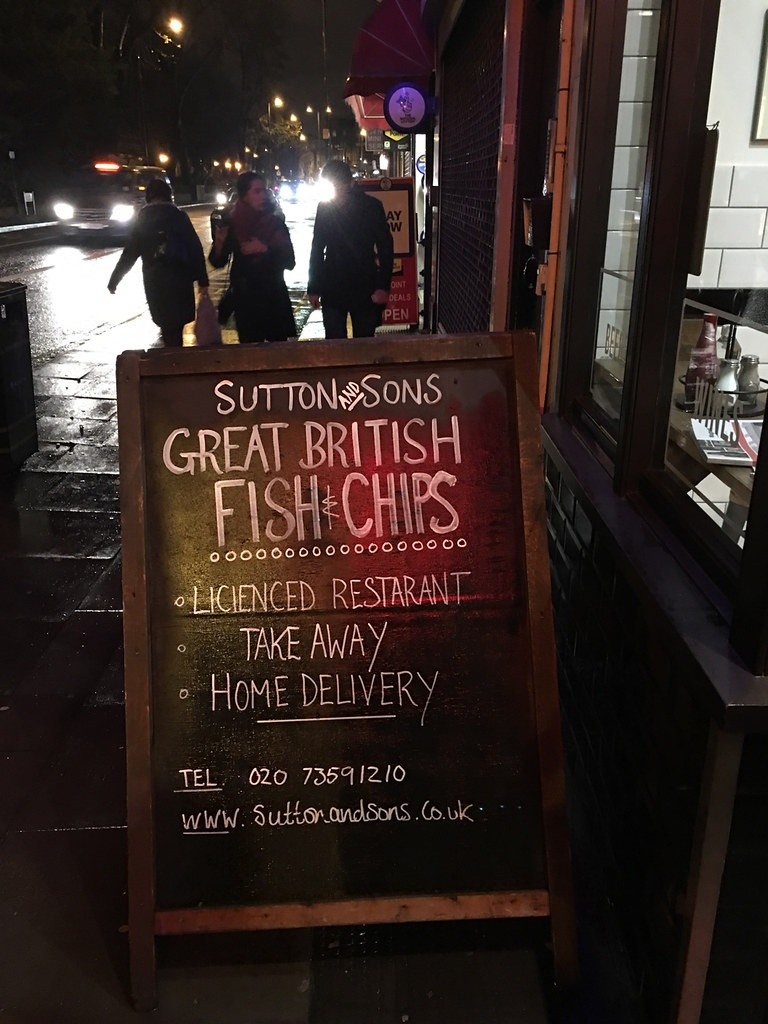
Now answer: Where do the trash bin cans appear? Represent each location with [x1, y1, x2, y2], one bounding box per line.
[0, 282, 37, 465]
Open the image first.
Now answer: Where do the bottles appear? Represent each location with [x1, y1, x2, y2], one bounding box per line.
[682, 313, 761, 412]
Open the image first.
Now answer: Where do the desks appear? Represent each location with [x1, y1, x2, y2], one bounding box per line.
[594, 288, 768, 543]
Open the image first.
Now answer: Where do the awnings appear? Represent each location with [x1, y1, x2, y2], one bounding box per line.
[342, 0, 436, 131]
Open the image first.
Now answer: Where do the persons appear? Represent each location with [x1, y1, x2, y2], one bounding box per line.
[307, 159, 395, 339]
[108, 179, 210, 347]
[208, 171, 298, 342]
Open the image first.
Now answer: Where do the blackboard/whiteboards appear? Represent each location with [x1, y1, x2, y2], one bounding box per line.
[117, 331, 586, 932]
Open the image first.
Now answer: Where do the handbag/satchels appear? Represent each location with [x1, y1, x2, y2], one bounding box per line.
[195, 293, 222, 347]
[217, 289, 237, 330]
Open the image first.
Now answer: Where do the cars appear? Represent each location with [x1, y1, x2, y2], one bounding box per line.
[52, 160, 175, 244]
[216, 171, 279, 206]
[272, 178, 306, 203]
[359, 176, 412, 190]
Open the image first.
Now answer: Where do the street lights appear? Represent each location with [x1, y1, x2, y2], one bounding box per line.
[267, 94, 284, 181]
[305, 103, 333, 172]
[138, 17, 187, 166]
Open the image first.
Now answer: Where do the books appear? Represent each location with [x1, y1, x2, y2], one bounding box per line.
[689, 418, 764, 466]
